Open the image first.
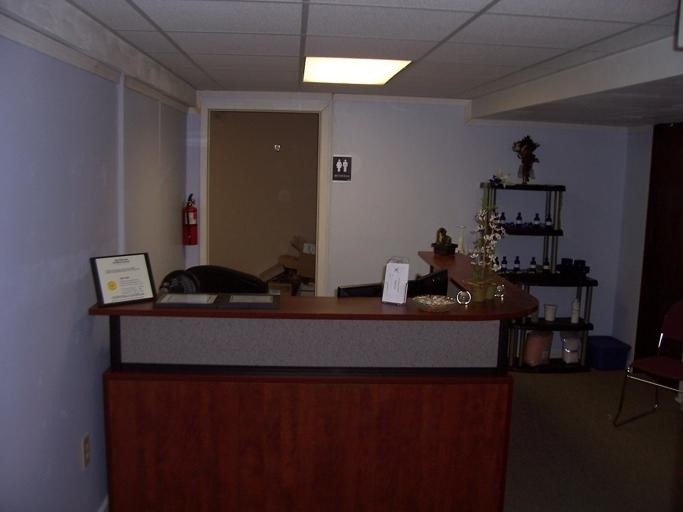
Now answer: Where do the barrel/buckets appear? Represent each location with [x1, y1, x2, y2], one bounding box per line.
[563, 338, 582, 363]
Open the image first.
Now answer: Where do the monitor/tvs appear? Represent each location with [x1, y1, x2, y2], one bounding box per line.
[414, 269, 449, 298]
[337, 280, 416, 298]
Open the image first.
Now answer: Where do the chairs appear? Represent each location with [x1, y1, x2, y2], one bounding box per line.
[613, 299, 683, 427]
[338, 269, 448, 298]
[187, 265, 268, 292]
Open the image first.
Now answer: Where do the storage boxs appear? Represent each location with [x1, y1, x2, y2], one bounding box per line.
[585, 335, 632, 370]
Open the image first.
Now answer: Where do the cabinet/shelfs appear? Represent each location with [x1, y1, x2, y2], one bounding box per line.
[480, 181, 598, 373]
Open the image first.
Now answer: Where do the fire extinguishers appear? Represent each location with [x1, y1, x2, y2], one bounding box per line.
[182, 193, 198, 246]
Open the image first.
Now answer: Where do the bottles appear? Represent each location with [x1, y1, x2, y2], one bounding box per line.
[530, 257, 536, 270]
[546, 214, 552, 226]
[544, 257, 549, 269]
[516, 212, 522, 226]
[500, 212, 505, 226]
[571, 297, 580, 324]
[514, 256, 520, 270]
[501, 255, 507, 272]
[534, 213, 540, 226]
